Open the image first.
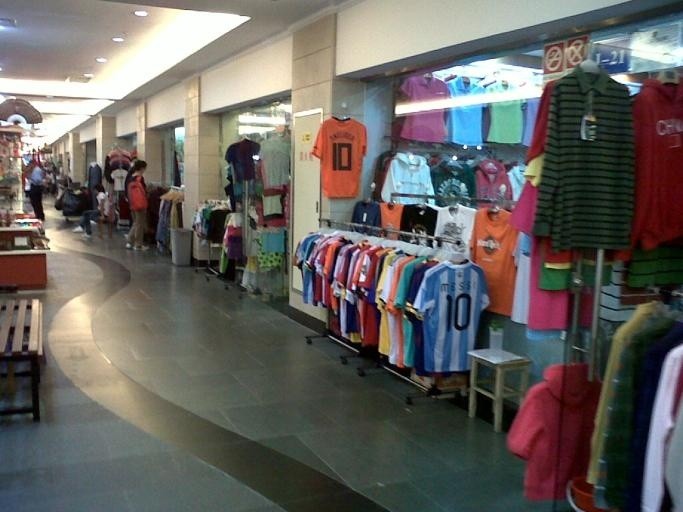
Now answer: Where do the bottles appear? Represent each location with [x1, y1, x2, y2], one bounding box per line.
[489, 320, 504, 357]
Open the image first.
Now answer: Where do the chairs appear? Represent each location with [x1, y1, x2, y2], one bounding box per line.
[96, 191, 114, 238]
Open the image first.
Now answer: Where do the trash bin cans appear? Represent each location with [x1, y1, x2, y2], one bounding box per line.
[170, 226, 193, 266]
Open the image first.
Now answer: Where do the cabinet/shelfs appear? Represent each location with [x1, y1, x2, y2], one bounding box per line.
[0, 226, 47, 290]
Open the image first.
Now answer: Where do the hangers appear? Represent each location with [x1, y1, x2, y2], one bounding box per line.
[304, 217, 477, 272]
[564, 40, 683, 94]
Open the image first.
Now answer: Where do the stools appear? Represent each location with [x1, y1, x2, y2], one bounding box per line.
[468, 348, 533, 433]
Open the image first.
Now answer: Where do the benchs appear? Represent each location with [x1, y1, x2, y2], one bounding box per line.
[1, 298, 46, 422]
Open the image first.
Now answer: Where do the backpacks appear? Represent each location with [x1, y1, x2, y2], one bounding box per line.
[127, 175, 149, 212]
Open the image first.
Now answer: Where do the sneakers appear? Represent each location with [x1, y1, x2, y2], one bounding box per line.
[81, 232, 92, 240]
[72, 225, 84, 233]
[125, 241, 149, 251]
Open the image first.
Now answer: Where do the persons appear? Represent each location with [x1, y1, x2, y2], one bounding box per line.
[72, 184, 112, 238]
[43, 161, 64, 197]
[29, 160, 44, 220]
[125, 157, 149, 251]
